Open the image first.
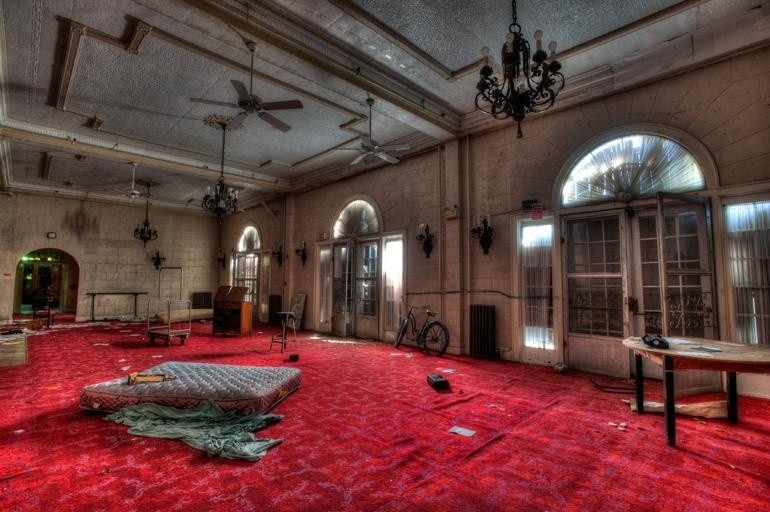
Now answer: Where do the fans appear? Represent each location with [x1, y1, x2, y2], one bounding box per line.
[334, 97, 410, 166]
[112, 163, 152, 203]
[189, 41, 304, 132]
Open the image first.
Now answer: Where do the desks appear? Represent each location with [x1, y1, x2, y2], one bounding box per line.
[86, 292, 148, 321]
[622, 335, 770, 447]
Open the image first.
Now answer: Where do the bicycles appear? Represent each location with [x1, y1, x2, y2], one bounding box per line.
[393, 296, 450, 357]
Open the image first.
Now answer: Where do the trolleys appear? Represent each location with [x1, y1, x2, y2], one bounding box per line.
[144, 295, 193, 348]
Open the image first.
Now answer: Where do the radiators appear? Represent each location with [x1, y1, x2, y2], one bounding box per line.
[469, 305, 496, 360]
[269, 295, 281, 325]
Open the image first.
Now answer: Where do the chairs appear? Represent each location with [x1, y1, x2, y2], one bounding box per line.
[33, 297, 55, 328]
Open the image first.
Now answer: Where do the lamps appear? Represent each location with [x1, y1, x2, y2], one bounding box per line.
[201, 115, 244, 225]
[272, 245, 282, 267]
[152, 251, 165, 270]
[218, 253, 225, 268]
[295, 242, 307, 265]
[416, 224, 434, 258]
[471, 216, 495, 255]
[133, 179, 161, 247]
[475, 1, 565, 139]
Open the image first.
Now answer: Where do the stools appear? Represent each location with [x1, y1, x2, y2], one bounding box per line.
[269, 311, 298, 353]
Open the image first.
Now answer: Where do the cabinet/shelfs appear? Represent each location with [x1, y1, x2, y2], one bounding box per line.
[212, 286, 253, 336]
[194, 293, 212, 309]
